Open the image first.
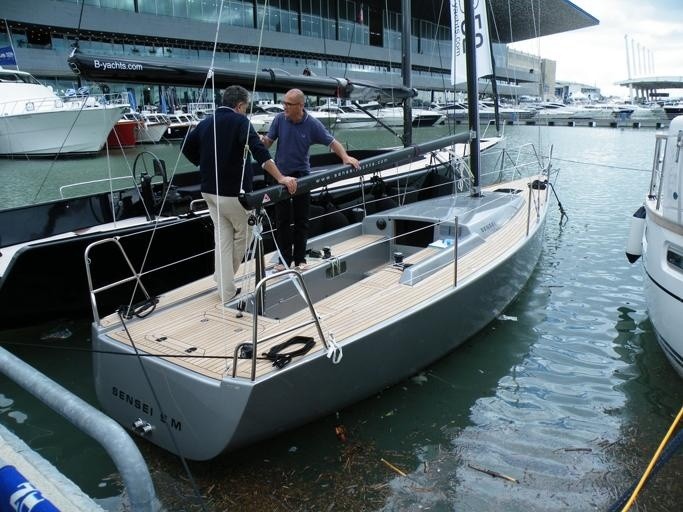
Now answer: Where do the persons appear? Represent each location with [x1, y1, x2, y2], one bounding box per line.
[257, 88, 361, 272]
[179, 84, 297, 306]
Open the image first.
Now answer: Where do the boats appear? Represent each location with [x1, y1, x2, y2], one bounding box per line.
[624, 114, 683, 381]
[80, 0, 566, 463]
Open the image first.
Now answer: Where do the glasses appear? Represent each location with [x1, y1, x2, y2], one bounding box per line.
[282, 101, 300, 109]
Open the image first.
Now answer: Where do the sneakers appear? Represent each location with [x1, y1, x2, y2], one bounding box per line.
[272, 262, 309, 273]
[236, 288, 241, 296]
